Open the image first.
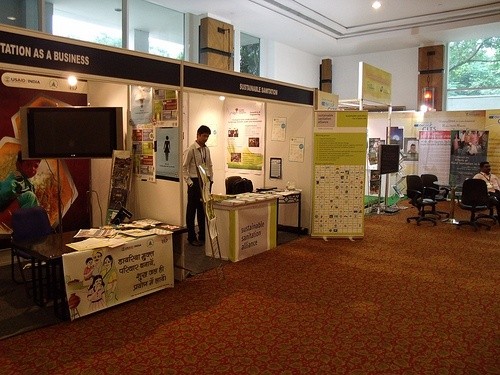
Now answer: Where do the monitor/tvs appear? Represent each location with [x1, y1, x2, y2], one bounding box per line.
[379, 144, 400, 174]
[19, 106, 124, 160]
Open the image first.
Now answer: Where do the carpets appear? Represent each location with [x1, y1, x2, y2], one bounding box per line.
[364, 195, 400, 207]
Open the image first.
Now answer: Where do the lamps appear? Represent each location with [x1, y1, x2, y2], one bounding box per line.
[420, 88, 434, 109]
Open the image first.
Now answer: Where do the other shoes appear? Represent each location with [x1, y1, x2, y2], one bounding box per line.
[188, 240, 202, 246]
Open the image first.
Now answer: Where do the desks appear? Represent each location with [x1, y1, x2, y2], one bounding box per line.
[15, 217, 189, 318]
[433, 180, 463, 225]
[205, 189, 302, 262]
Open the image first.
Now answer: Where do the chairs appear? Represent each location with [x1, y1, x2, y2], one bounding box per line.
[9, 206, 55, 299]
[224, 175, 241, 194]
[406, 174, 450, 227]
[458, 179, 499, 232]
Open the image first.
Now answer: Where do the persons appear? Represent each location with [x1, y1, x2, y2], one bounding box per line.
[454, 131, 489, 156]
[183, 126, 214, 246]
[472, 161, 500, 223]
[0, 151, 41, 213]
